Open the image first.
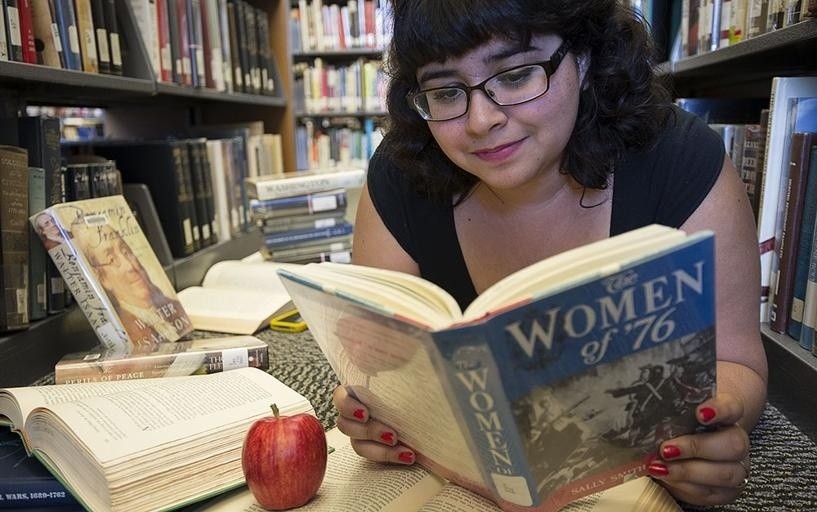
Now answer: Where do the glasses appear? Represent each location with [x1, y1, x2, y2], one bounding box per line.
[406, 37, 571, 122]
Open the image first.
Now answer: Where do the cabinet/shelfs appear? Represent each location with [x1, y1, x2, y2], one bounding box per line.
[616, 0, 816, 422]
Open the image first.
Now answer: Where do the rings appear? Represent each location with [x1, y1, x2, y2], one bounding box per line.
[732, 459, 750, 489]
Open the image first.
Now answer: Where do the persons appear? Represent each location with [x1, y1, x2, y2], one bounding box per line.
[330, 2, 767, 509]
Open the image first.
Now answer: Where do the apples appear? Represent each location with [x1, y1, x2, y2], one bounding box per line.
[242, 403, 327, 510]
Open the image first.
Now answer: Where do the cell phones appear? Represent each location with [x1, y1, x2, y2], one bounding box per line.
[270, 308, 309, 334]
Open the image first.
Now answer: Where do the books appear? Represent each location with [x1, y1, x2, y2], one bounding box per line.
[183, 426, 682, 512]
[629, 1, 817, 361]
[0, 1, 285, 98]
[0, 111, 406, 512]
[289, 0, 394, 114]
[272, 219, 720, 512]
